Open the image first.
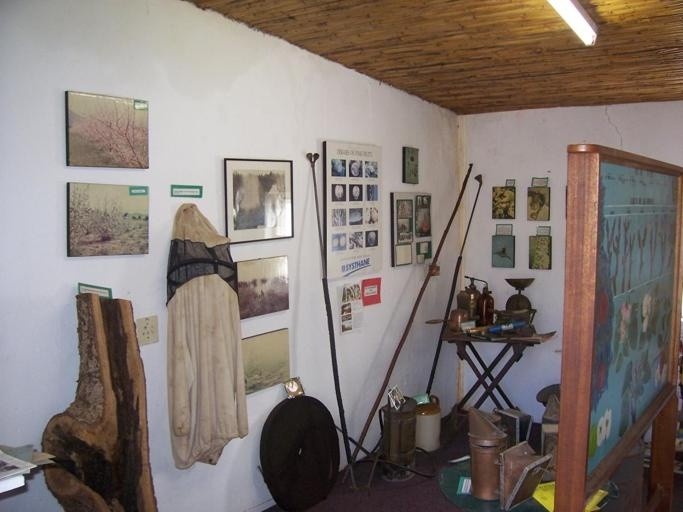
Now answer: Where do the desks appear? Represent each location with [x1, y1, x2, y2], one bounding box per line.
[441, 325, 553, 446]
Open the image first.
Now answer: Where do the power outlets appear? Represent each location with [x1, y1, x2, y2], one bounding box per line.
[135, 315, 160, 346]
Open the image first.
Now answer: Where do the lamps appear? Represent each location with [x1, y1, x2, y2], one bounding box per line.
[547, 0, 597, 48]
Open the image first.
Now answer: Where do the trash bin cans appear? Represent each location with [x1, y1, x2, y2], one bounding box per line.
[468, 406, 507, 500]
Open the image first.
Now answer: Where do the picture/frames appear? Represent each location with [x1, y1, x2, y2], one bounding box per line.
[235, 255, 288, 319]
[506, 454, 557, 509]
[390, 191, 431, 265]
[499, 441, 534, 508]
[492, 234, 515, 268]
[242, 328, 290, 395]
[402, 146, 419, 182]
[555, 143, 683, 494]
[492, 186, 515, 219]
[529, 236, 552, 270]
[224, 158, 294, 244]
[65, 91, 149, 169]
[495, 408, 519, 447]
[67, 182, 149, 257]
[527, 187, 550, 221]
[323, 140, 382, 278]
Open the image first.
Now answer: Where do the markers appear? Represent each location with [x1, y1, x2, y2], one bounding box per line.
[488, 321, 526, 332]
